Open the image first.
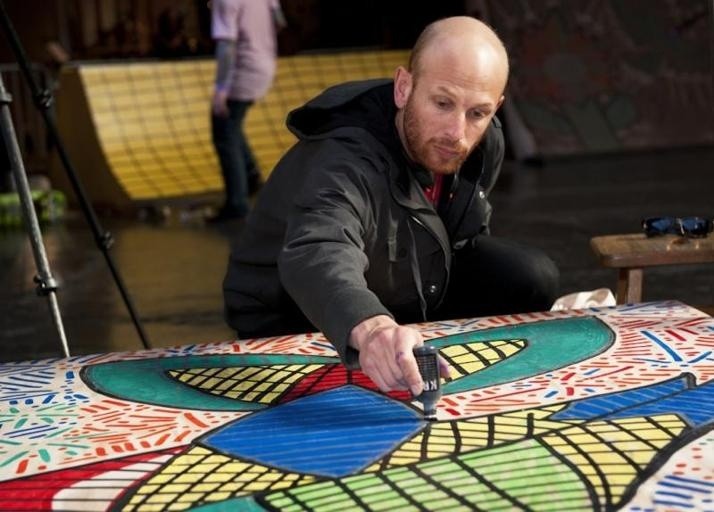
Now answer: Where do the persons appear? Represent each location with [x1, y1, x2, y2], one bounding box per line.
[221, 14, 561, 400]
[198, 0, 288, 225]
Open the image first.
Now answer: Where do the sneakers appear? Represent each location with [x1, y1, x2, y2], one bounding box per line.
[205, 202, 248, 224]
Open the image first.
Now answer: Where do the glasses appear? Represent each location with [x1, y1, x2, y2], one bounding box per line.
[642, 216, 712, 238]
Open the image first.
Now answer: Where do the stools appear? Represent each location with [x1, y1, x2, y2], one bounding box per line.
[592, 233, 713, 302]
